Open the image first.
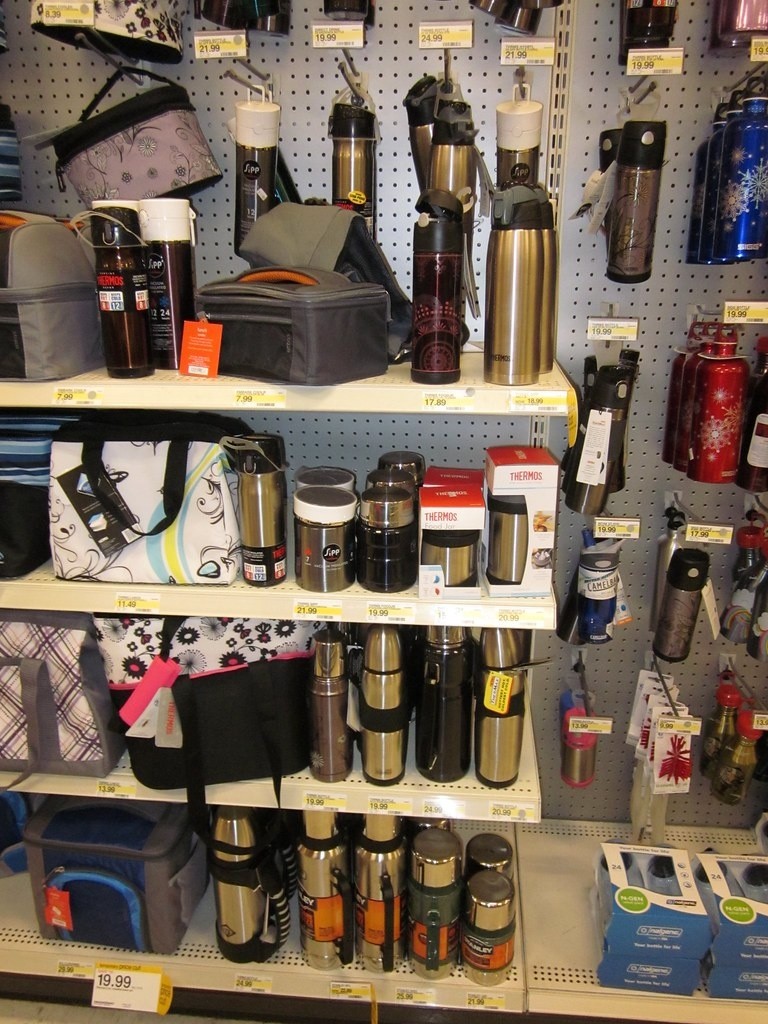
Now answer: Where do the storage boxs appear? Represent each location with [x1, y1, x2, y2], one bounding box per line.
[591, 839, 768, 1003]
[417, 447, 558, 598]
[22, 800, 212, 954]
[193, 269, 388, 386]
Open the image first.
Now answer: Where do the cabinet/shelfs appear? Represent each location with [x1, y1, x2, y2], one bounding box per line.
[0, 341, 576, 1024]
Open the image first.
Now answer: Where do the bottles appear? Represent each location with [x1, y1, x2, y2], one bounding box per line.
[91, 198, 198, 378]
[699, 669, 763, 806]
[228, 85, 303, 259]
[718, 524, 768, 664]
[685, 76, 768, 265]
[595, 120, 667, 283]
[555, 535, 626, 644]
[497, 84, 543, 186]
[560, 348, 642, 516]
[737, 337, 768, 493]
[411, 190, 464, 385]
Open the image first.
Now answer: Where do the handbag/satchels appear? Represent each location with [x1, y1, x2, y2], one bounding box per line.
[193, 266, 392, 385]
[22, 793, 210, 955]
[30, 0, 190, 66]
[49, 409, 255, 588]
[0, 607, 129, 778]
[92, 609, 335, 791]
[52, 65, 225, 211]
[0, 208, 107, 382]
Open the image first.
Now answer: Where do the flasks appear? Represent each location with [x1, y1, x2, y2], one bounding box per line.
[560, 691, 598, 788]
[648, 506, 709, 662]
[484, 184, 556, 385]
[405, 75, 478, 193]
[234, 433, 529, 594]
[410, 832, 520, 987]
[309, 624, 527, 788]
[210, 805, 408, 973]
[661, 322, 751, 485]
[328, 103, 376, 243]
